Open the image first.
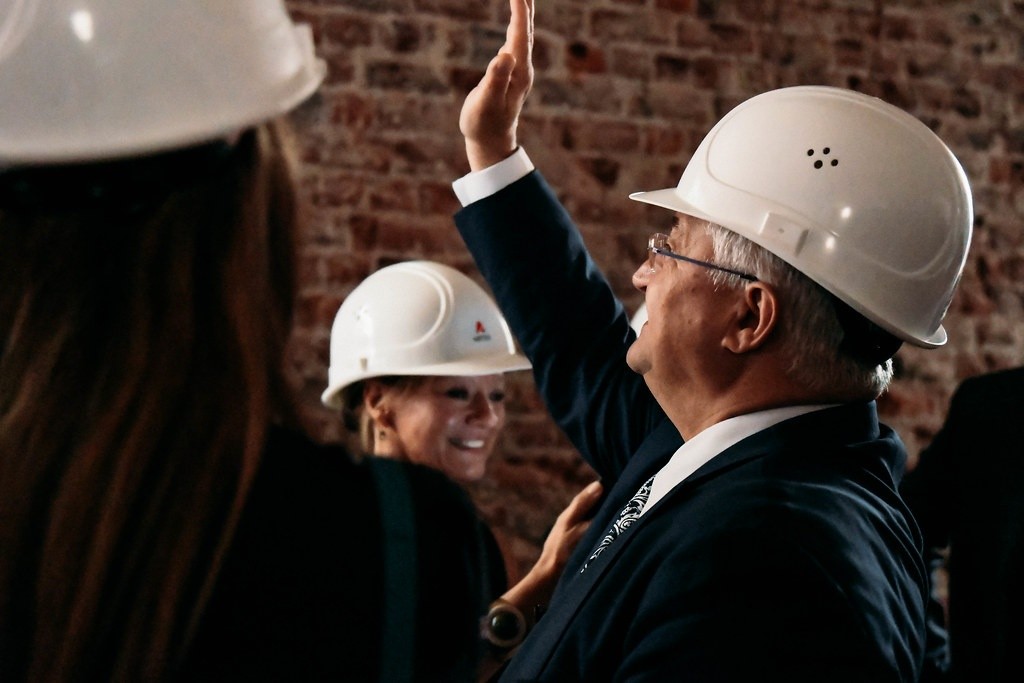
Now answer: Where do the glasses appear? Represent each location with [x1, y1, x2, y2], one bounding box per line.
[647, 232, 760, 282]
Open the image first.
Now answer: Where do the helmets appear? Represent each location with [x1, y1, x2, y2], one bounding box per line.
[320, 260, 534, 411]
[629, 85, 974, 350]
[0, 0, 328, 165]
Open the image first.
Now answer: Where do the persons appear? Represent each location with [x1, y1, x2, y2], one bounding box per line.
[904, 367, 1022, 683]
[0, 0, 599, 683]
[451, 0, 975, 682]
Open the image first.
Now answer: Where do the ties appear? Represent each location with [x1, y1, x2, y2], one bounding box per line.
[575, 473, 656, 579]
